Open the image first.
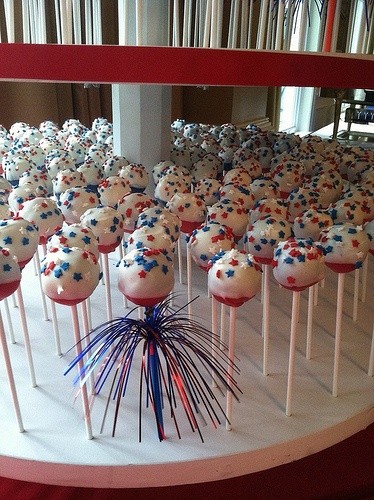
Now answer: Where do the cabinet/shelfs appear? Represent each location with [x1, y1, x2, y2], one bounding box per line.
[182, 85, 281, 133]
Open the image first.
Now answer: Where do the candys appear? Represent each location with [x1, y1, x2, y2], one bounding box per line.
[0, 117, 374, 308]
